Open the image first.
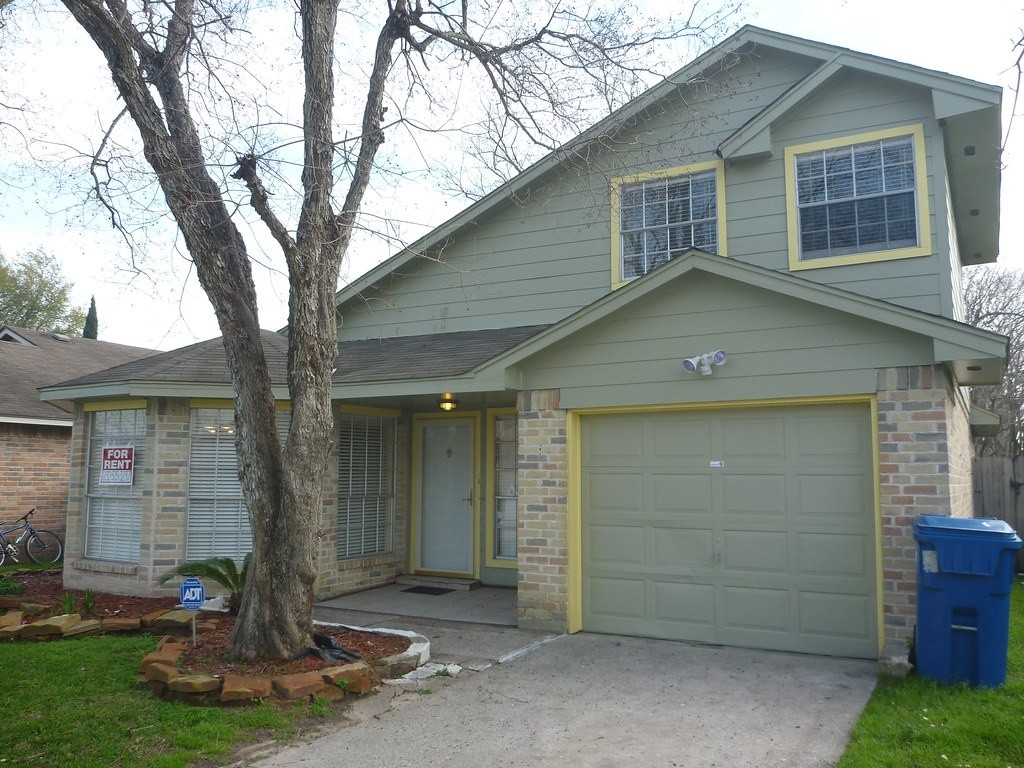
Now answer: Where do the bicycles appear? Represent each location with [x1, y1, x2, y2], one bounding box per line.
[0, 508, 62, 568]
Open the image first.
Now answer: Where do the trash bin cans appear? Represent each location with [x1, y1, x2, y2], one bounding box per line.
[910, 513, 1023, 689]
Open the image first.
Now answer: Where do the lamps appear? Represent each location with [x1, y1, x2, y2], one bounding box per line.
[439, 401, 457, 413]
[682, 350, 727, 376]
[204, 418, 236, 434]
